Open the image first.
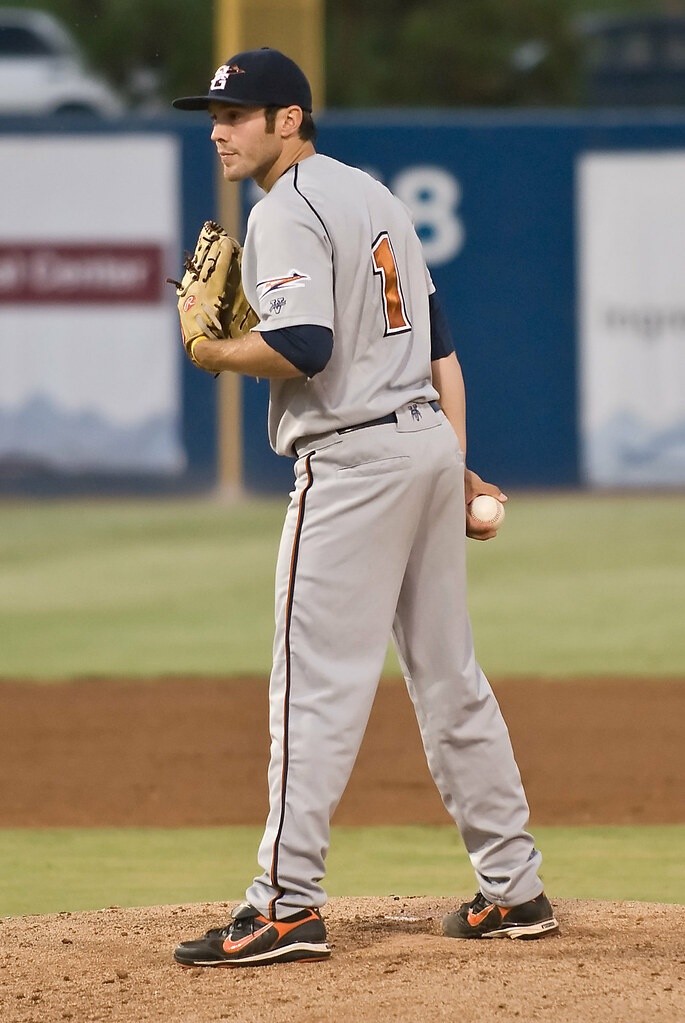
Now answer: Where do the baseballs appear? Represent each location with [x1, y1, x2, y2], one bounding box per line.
[468, 494, 505, 532]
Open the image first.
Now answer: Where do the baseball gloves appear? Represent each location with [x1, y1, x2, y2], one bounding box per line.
[176, 220, 261, 378]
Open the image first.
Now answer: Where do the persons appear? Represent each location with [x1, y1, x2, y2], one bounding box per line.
[172, 47, 561, 970]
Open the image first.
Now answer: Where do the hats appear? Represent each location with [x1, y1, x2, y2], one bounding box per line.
[169, 46, 314, 114]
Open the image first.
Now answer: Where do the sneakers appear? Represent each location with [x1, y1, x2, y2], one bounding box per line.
[440, 888, 559, 940]
[173, 899, 331, 968]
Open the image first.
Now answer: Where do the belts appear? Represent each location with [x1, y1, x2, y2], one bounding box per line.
[289, 399, 442, 458]
